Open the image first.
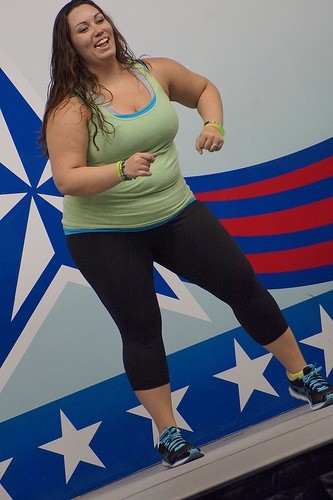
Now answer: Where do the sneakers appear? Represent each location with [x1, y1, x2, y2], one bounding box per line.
[157, 426, 204, 467]
[287, 363, 333, 411]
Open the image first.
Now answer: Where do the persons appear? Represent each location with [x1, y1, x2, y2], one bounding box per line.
[35, 0, 333, 467]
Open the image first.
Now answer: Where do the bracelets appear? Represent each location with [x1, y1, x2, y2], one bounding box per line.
[203, 120, 225, 136]
[116, 158, 139, 182]
